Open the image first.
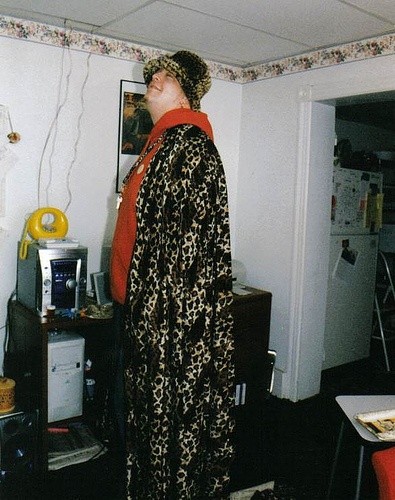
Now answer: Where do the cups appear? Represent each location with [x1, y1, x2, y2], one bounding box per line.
[0, 378, 16, 413]
[86, 379, 96, 397]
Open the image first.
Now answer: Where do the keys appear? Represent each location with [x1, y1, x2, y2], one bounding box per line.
[115, 195, 123, 209]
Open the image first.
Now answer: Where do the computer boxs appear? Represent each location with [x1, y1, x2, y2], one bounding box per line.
[47, 332, 85, 421]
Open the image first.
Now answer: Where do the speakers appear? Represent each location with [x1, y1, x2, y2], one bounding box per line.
[0, 410, 44, 500]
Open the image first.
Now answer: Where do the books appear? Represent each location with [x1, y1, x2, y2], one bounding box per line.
[235, 382, 246, 405]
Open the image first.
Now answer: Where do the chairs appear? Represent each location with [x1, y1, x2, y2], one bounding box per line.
[370, 446, 395, 500]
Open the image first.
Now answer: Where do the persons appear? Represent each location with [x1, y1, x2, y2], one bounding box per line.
[109, 50, 236, 500]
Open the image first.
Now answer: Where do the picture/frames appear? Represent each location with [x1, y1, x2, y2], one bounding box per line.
[117, 78, 153, 196]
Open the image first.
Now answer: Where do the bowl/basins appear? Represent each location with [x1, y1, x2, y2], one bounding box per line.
[374, 151, 395, 159]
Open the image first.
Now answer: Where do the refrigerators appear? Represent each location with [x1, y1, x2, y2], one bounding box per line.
[322, 166, 383, 368]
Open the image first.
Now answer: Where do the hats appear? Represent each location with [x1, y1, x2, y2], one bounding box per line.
[142, 50, 213, 110]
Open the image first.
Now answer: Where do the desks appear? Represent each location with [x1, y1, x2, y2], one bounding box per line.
[8, 284, 275, 484]
[325, 385, 395, 500]
